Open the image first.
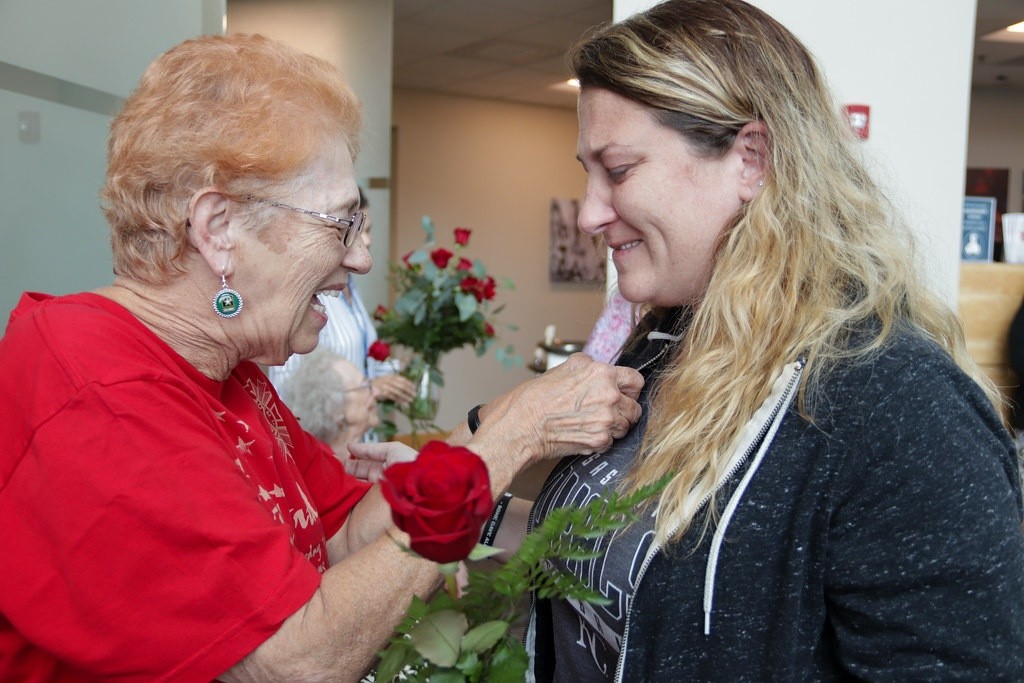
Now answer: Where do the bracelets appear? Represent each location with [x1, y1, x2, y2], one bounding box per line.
[467, 404, 481, 435]
[479, 492, 514, 559]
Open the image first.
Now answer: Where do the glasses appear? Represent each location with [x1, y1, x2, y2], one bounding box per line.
[184, 192, 366, 247]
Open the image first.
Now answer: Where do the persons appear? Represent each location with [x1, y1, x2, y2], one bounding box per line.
[279, 351, 380, 465]
[265, 187, 417, 443]
[337, 1, 1024, 683]
[0, 35, 647, 683]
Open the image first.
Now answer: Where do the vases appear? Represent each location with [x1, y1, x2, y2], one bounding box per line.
[397, 363, 446, 421]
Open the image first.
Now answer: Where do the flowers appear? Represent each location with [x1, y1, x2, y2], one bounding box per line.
[373, 440, 680, 683]
[362, 215, 525, 420]
[366, 337, 400, 442]
